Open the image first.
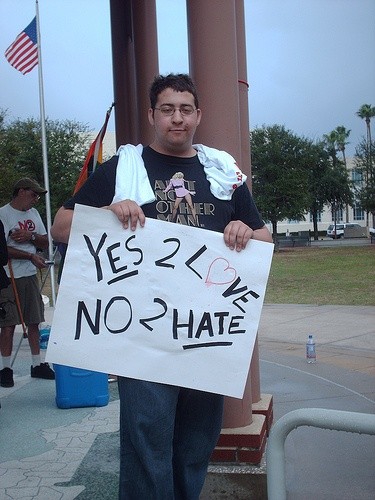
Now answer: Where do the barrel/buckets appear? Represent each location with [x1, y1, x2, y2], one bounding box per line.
[52, 364, 110, 409]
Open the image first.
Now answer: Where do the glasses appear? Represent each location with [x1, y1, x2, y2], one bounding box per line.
[31, 193, 41, 200]
[154, 106, 196, 117]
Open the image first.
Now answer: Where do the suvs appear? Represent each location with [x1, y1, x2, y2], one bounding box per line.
[326, 223, 360, 239]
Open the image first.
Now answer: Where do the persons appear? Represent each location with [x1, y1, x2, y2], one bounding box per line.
[50, 73, 273, 500]
[0, 219, 11, 289]
[0, 177, 55, 388]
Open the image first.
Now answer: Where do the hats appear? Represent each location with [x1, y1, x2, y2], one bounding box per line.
[15, 177, 47, 196]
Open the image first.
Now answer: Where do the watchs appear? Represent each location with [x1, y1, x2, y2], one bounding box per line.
[30, 232, 35, 242]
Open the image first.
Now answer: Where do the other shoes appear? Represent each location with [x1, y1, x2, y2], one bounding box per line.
[31, 362, 55, 380]
[0, 367, 14, 388]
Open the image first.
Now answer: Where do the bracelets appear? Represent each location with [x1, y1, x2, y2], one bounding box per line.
[29, 253, 34, 260]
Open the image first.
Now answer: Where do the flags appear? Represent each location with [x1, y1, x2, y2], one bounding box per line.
[4, 16, 39, 76]
[57, 110, 111, 287]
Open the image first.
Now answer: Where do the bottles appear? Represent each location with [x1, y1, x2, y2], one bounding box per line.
[306, 336, 316, 364]
[38, 320, 51, 363]
